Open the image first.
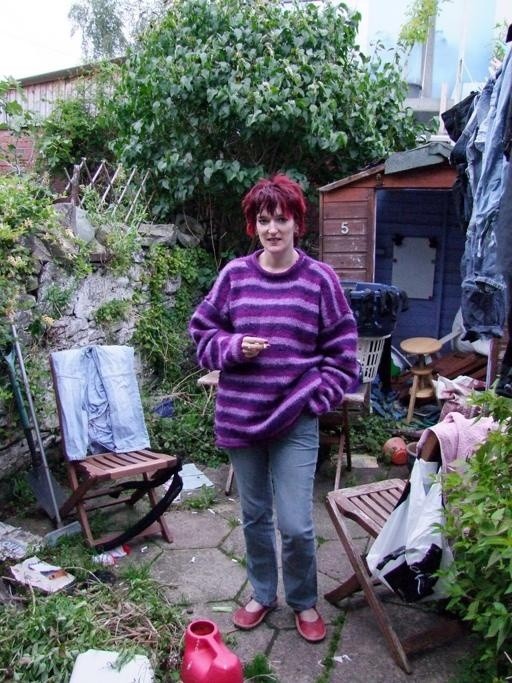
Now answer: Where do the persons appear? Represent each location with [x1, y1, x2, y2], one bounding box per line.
[188, 174, 362, 646]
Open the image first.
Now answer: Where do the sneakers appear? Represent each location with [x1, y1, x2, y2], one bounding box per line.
[294, 607, 327, 641]
[233, 599, 274, 630]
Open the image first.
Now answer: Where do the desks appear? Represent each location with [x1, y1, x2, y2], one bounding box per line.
[398, 336, 444, 427]
[195, 367, 374, 495]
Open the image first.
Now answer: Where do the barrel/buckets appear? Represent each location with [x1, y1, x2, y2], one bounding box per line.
[179, 619, 244, 682]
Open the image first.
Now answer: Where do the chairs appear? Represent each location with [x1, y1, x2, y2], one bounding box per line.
[323, 414, 507, 675]
[48, 341, 180, 554]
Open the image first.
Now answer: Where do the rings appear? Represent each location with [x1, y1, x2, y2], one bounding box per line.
[247, 344, 252, 351]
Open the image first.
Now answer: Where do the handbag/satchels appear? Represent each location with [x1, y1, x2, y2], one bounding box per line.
[366, 458, 462, 603]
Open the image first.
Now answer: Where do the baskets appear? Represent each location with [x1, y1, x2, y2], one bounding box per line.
[357, 334, 394, 384]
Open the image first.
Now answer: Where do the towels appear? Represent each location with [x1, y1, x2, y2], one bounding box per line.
[413, 409, 511, 599]
